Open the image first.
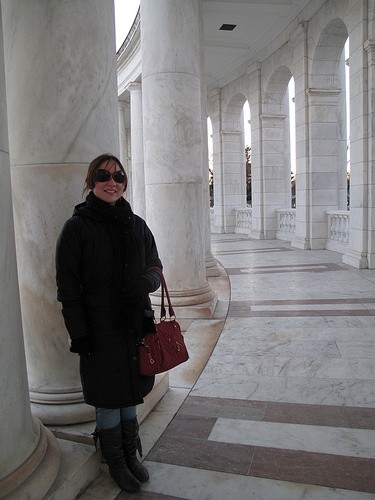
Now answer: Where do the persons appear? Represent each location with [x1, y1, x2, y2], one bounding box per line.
[55, 154, 163, 491]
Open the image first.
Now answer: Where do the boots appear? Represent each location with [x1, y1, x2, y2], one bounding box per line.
[91, 422, 142, 491]
[120, 415, 149, 482]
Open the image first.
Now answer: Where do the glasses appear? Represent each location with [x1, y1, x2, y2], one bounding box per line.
[95, 169, 125, 183]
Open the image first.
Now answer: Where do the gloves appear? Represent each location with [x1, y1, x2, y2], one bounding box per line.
[70, 333, 95, 355]
[124, 279, 148, 305]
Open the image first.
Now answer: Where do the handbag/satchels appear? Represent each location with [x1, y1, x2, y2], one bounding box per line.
[138, 267, 188, 376]
